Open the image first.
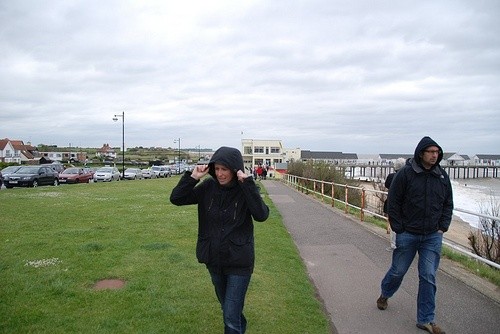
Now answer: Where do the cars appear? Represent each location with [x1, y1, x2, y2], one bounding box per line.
[124, 168, 143, 181]
[3, 166, 62, 189]
[0, 165, 23, 189]
[57, 167, 96, 184]
[93, 167, 122, 183]
[141, 161, 194, 179]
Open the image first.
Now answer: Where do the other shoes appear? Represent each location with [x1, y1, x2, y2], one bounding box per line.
[377, 295, 389, 310]
[416, 322, 446, 334]
[386, 247, 394, 252]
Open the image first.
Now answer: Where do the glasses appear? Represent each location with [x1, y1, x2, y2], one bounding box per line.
[424, 149, 441, 154]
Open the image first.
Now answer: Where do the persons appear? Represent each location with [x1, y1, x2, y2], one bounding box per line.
[172, 146, 269, 334]
[383, 157, 406, 252]
[249, 164, 269, 180]
[377, 138, 454, 334]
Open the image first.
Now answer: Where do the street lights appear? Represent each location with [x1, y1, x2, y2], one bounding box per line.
[112, 112, 126, 181]
[173, 138, 180, 175]
